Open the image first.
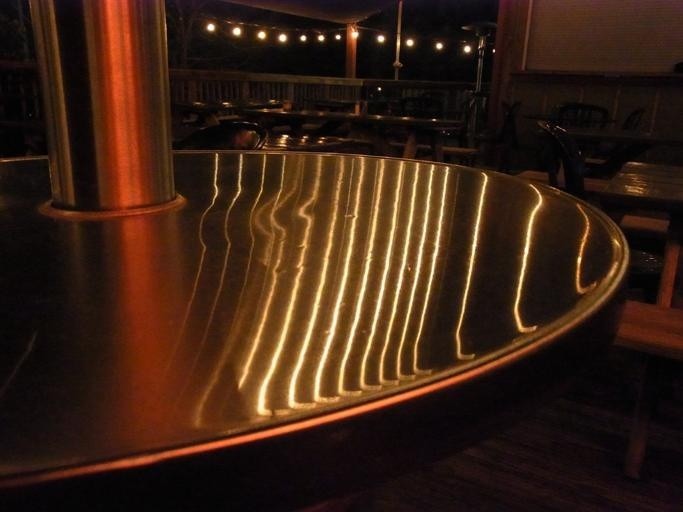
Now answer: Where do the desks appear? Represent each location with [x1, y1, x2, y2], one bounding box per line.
[0, 94, 482, 163]
[0, 148, 630, 490]
